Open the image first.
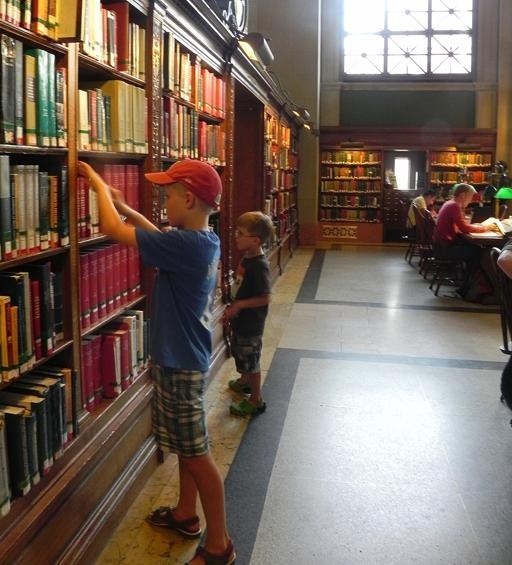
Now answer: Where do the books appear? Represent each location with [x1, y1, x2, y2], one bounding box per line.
[430, 150, 492, 208]
[264, 111, 298, 254]
[318, 148, 381, 222]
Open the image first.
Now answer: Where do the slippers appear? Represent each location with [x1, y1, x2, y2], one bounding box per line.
[229, 379, 250, 393]
[230, 399, 265, 415]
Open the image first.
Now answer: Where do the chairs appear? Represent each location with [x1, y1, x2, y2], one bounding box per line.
[394, 197, 512, 355]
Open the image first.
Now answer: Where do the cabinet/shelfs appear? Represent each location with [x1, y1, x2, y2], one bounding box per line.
[316, 144, 383, 224]
[0, 1, 303, 564]
[423, 147, 494, 222]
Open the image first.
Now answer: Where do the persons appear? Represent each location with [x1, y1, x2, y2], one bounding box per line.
[432, 183, 495, 302]
[497, 239, 512, 281]
[405, 191, 437, 239]
[224, 211, 273, 416]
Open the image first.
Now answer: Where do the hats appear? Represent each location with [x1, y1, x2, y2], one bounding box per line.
[144, 160, 222, 210]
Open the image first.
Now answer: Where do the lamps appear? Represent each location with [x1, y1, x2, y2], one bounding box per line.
[298, 105, 317, 138]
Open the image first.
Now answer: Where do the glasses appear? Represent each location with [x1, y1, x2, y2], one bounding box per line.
[235, 231, 257, 239]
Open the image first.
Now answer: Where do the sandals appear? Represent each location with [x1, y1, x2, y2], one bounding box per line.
[185, 537, 236, 565]
[146, 505, 202, 539]
